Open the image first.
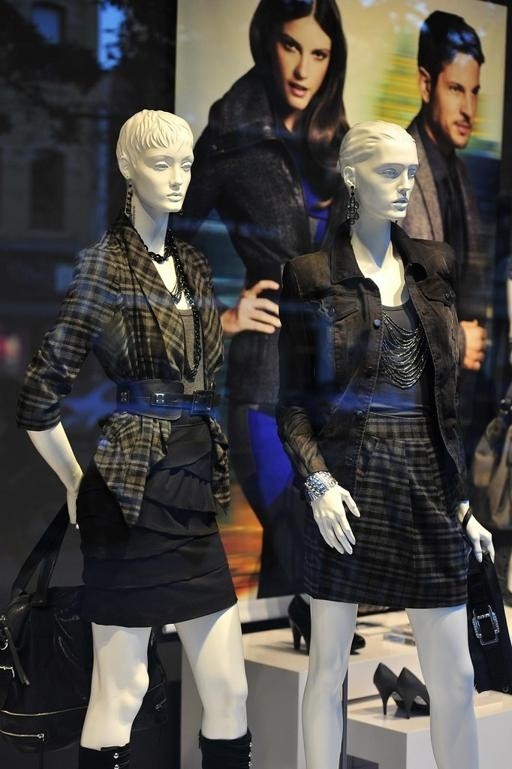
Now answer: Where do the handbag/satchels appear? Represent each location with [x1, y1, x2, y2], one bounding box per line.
[0, 501, 169, 754]
[468, 391, 512, 530]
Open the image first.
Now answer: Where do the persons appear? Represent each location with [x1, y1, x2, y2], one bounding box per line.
[273, 118, 496, 768]
[174, 0, 352, 600]
[403, 8, 511, 464]
[13, 107, 252, 769]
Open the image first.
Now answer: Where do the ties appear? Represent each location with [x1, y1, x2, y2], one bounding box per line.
[446, 169, 464, 265]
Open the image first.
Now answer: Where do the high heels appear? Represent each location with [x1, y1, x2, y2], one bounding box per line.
[395, 667, 429, 718]
[373, 662, 398, 714]
[288, 594, 366, 656]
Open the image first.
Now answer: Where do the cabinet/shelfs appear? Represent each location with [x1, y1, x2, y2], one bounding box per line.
[232, 604, 512, 769]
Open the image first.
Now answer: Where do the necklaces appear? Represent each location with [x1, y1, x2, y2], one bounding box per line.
[137, 229, 201, 382]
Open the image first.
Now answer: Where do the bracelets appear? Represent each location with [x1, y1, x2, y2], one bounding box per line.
[304, 471, 338, 506]
